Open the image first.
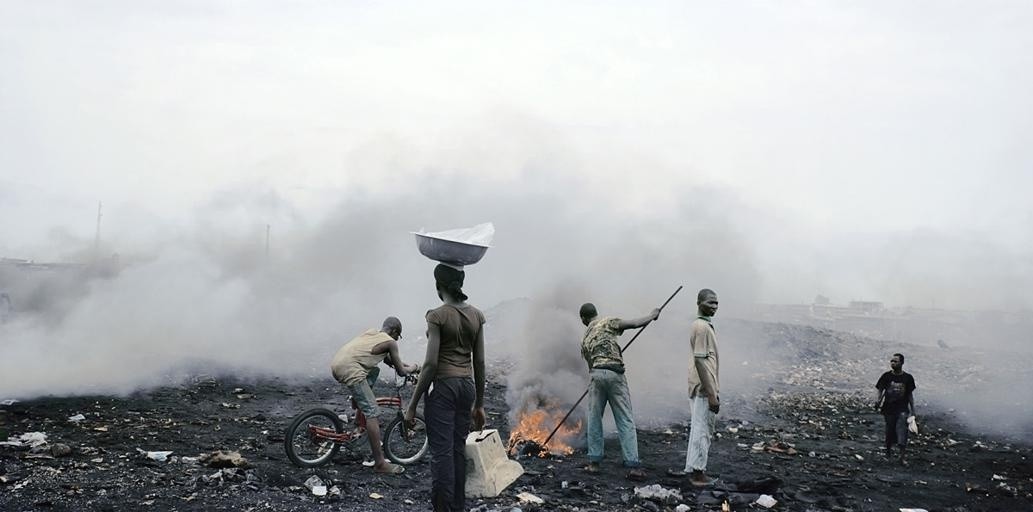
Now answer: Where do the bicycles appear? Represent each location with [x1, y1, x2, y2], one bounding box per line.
[283, 365, 430, 468]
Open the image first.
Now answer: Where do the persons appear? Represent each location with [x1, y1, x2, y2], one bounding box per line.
[684, 288, 721, 487]
[579, 302, 660, 481]
[874, 352, 918, 469]
[330, 316, 418, 475]
[402, 263, 488, 512]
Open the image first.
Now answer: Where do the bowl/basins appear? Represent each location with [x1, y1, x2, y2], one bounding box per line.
[408, 231, 492, 266]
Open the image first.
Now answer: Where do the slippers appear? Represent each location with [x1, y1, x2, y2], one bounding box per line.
[373, 462, 405, 474]
[581, 462, 719, 486]
